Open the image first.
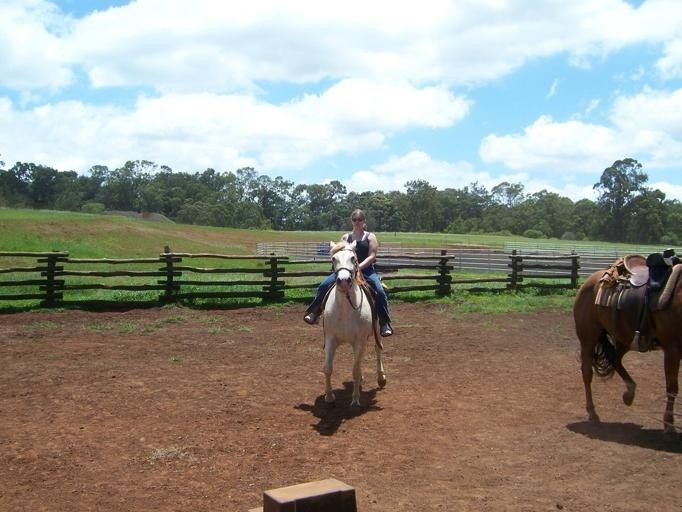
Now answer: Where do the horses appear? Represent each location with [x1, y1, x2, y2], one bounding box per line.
[574, 269, 682, 442]
[322, 239, 386, 408]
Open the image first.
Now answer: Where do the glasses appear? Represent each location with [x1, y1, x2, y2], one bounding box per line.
[351, 218, 365, 222]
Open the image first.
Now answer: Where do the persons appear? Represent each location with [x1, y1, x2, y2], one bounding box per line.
[302, 209, 394, 337]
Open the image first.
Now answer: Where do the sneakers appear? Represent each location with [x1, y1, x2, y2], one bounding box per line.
[381, 326, 392, 336]
[305, 313, 321, 325]
